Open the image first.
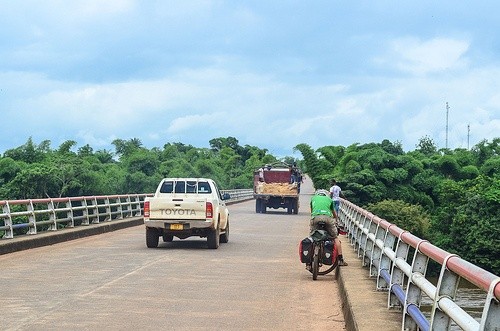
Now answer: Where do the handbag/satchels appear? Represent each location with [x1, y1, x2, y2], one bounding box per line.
[299, 238, 314, 263]
[320, 238, 337, 265]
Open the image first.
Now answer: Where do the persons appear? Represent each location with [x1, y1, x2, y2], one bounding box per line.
[310, 179, 349, 266]
[292, 162, 304, 183]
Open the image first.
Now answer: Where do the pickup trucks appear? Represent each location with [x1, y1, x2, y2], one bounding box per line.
[143, 177, 231, 249]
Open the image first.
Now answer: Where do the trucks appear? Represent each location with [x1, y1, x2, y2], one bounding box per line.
[252, 162, 303, 215]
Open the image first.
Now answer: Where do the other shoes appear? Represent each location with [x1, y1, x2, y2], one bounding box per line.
[337, 259, 348, 266]
[305, 263, 312, 271]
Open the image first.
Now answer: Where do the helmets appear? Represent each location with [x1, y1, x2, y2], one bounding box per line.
[315, 188, 329, 197]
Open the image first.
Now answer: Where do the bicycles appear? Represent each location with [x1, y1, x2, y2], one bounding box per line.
[306, 216, 340, 280]
